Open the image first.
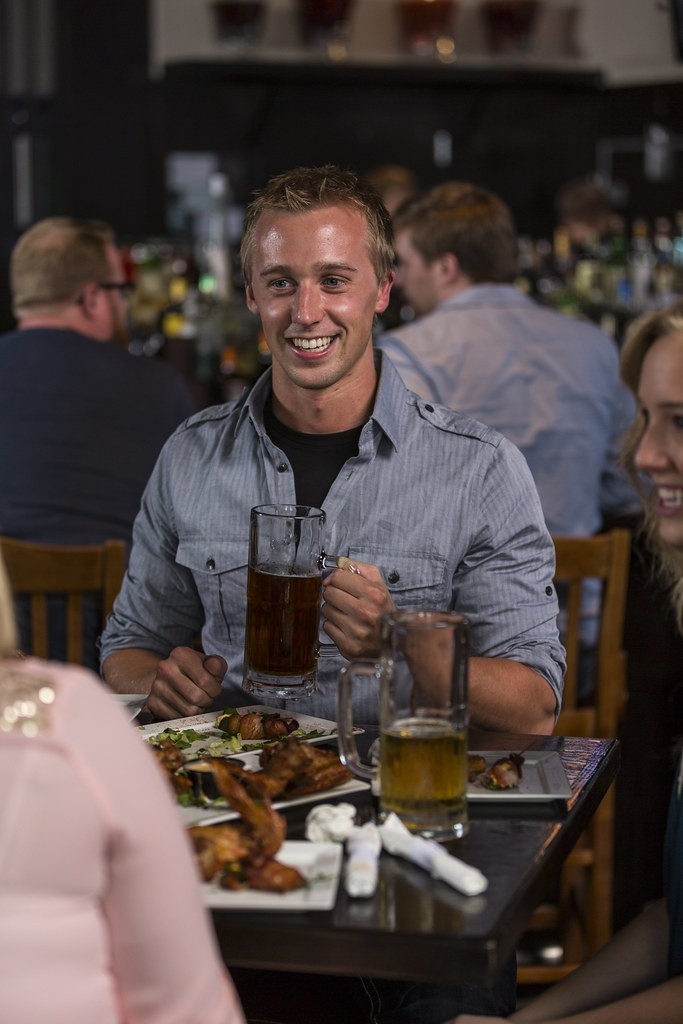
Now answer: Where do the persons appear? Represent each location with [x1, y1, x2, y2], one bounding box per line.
[362, 171, 435, 324]
[374, 182, 653, 715]
[440, 306, 683, 1024]
[2, 556, 247, 1024]
[112, 161, 570, 740]
[1, 218, 213, 674]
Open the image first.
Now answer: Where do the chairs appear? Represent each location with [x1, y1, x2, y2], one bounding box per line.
[517, 522, 630, 984]
[0, 538, 129, 670]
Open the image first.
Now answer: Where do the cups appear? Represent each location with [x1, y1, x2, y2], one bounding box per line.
[243, 505, 361, 700]
[337, 609, 470, 844]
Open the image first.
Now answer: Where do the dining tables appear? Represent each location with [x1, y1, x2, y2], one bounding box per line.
[206, 721, 622, 1015]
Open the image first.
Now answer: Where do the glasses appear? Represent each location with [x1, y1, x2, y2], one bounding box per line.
[77, 280, 136, 308]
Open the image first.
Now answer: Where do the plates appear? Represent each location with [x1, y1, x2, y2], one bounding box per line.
[112, 693, 149, 723]
[169, 749, 370, 830]
[132, 705, 364, 776]
[370, 751, 574, 803]
[203, 839, 344, 913]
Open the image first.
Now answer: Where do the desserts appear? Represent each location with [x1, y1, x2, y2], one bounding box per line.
[468, 740, 538, 792]
